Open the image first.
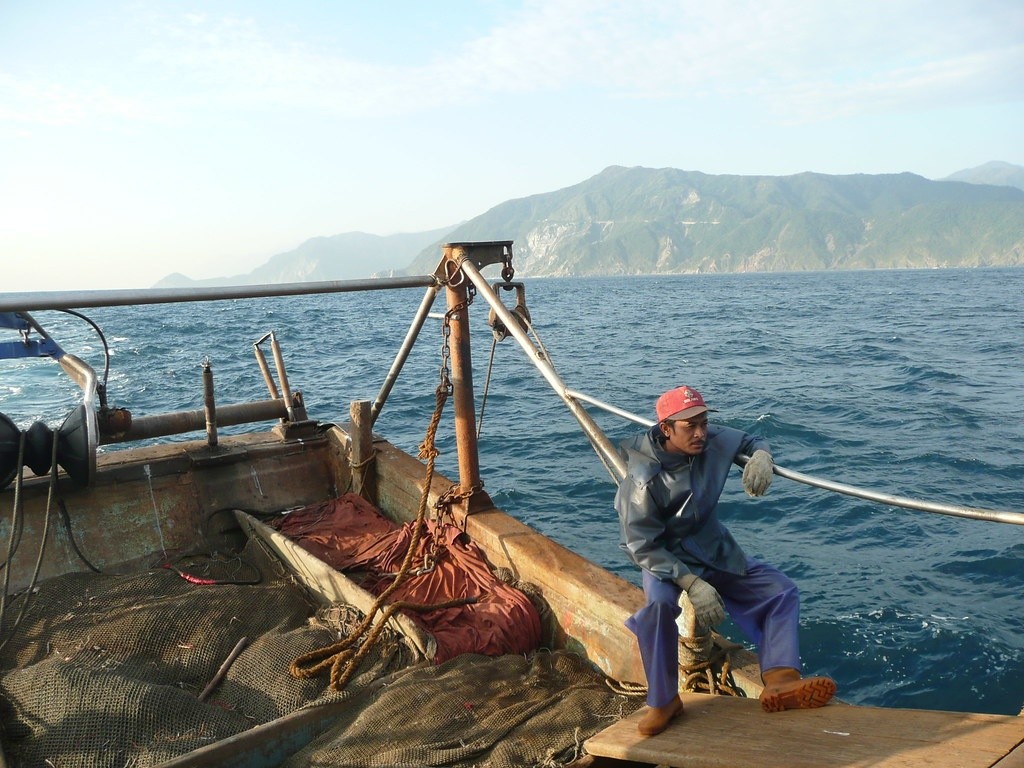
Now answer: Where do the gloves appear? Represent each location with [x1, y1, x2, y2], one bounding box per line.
[742, 449, 774, 498]
[671, 573, 725, 628]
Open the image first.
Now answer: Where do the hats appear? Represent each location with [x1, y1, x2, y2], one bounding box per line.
[656, 385, 719, 423]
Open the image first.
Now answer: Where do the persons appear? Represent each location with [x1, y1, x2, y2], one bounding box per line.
[613, 387, 838, 735]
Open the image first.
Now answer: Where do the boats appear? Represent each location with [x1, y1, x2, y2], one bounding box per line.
[0, 240, 1023, 766]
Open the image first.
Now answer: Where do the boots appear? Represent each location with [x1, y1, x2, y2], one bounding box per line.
[637, 694, 684, 738]
[759, 667, 835, 713]
